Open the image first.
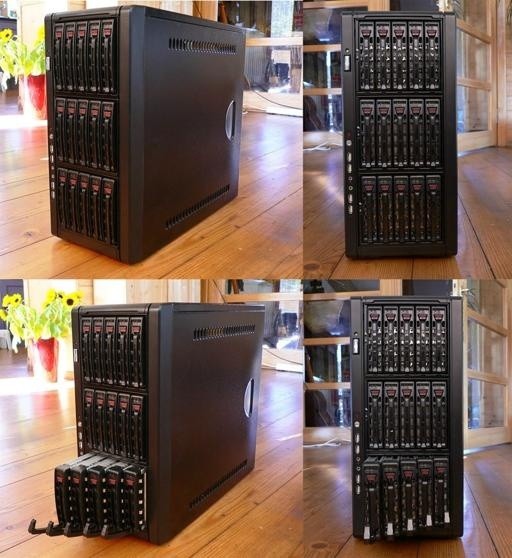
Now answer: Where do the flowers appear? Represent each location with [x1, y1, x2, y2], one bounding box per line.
[0, 25, 46, 80]
[0, 289, 85, 355]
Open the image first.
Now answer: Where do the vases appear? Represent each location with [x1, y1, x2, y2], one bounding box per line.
[28, 338, 60, 384]
[18, 73, 47, 120]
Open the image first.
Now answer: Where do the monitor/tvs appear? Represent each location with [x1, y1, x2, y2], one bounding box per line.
[0, 17, 18, 40]
